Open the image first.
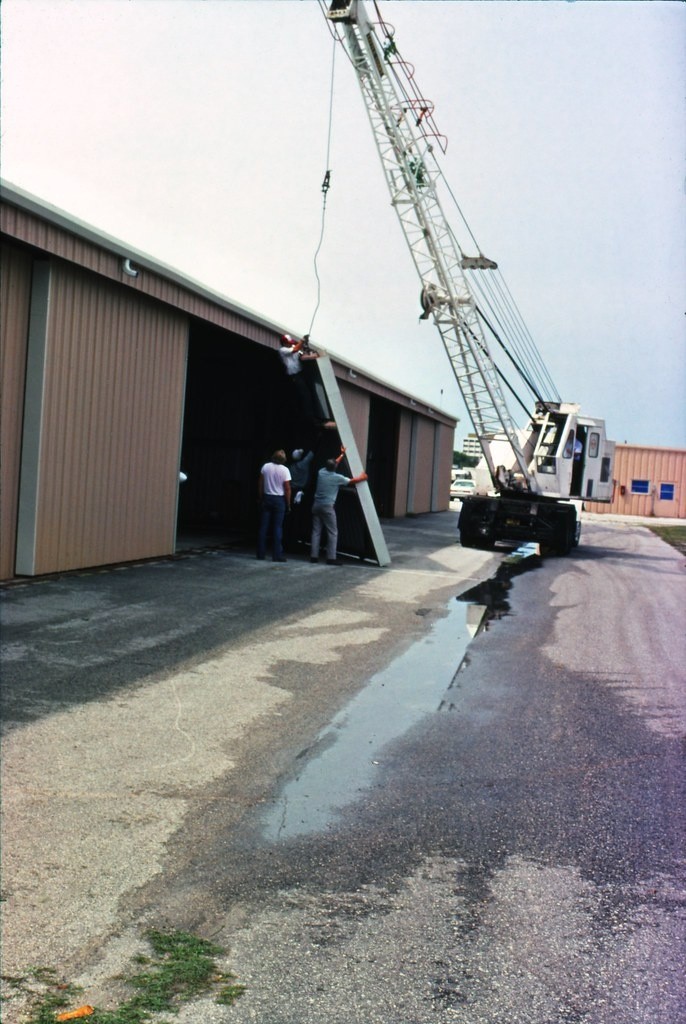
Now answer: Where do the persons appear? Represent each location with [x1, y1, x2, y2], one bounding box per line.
[310, 445, 368, 567]
[258, 449, 292, 563]
[562, 428, 582, 484]
[279, 334, 335, 427]
[290, 448, 319, 552]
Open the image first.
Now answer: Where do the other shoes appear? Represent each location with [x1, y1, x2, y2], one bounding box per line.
[311, 556, 343, 566]
[256, 552, 286, 562]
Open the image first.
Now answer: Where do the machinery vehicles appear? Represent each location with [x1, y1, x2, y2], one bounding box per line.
[321, 0, 616, 554]
